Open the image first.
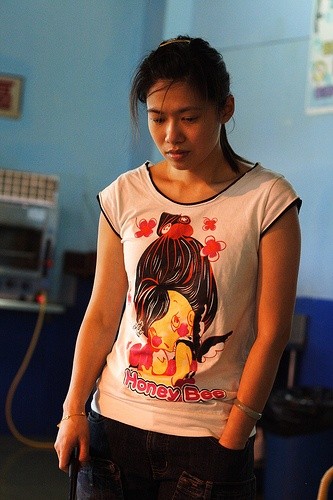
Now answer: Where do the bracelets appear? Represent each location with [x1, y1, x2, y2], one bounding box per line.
[233, 398, 263, 420]
[57, 413, 86, 427]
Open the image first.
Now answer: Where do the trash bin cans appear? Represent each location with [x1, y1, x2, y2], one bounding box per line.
[255, 386, 333, 500]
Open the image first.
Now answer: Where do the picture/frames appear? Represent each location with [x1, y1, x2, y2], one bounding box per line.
[0, 72, 26, 120]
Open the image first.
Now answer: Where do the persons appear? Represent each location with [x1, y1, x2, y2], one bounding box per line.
[54, 34, 304, 500]
